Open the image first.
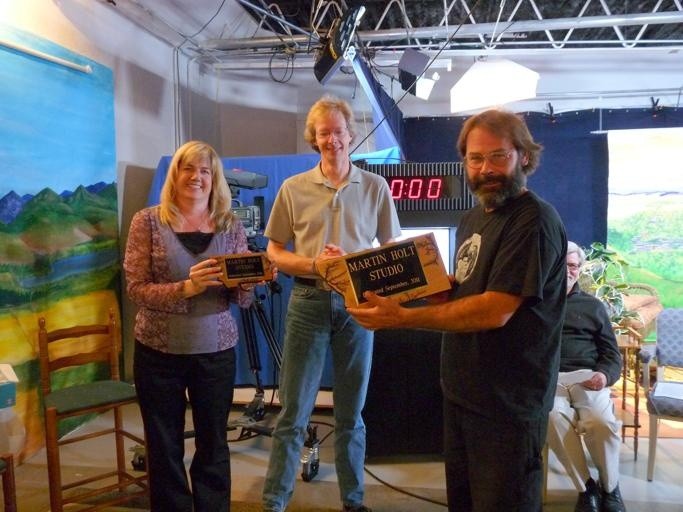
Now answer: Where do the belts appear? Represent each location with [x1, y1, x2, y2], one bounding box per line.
[293, 275, 333, 291]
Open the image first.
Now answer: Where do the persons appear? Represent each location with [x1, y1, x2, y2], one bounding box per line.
[346, 111, 569, 511]
[547, 238, 628, 511]
[122, 141, 259, 511]
[256, 94, 403, 511]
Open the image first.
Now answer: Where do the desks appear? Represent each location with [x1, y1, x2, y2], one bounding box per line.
[611, 327, 644, 464]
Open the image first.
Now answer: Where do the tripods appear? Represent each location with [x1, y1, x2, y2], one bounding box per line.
[130, 244, 321, 482]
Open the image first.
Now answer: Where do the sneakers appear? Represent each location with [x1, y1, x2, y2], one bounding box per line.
[343, 504, 372, 512]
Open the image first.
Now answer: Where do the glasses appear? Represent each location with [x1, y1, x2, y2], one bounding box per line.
[318, 126, 349, 139]
[463, 150, 512, 170]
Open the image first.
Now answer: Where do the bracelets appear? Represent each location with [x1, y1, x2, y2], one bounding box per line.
[309, 256, 317, 277]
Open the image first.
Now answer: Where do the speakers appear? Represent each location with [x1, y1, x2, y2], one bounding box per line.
[361, 298, 443, 456]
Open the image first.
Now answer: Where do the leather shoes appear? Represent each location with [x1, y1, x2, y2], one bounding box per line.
[573, 476, 600, 512]
[599, 480, 626, 512]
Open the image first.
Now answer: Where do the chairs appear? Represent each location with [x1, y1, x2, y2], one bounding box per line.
[640, 307, 683, 482]
[39, 306, 149, 512]
